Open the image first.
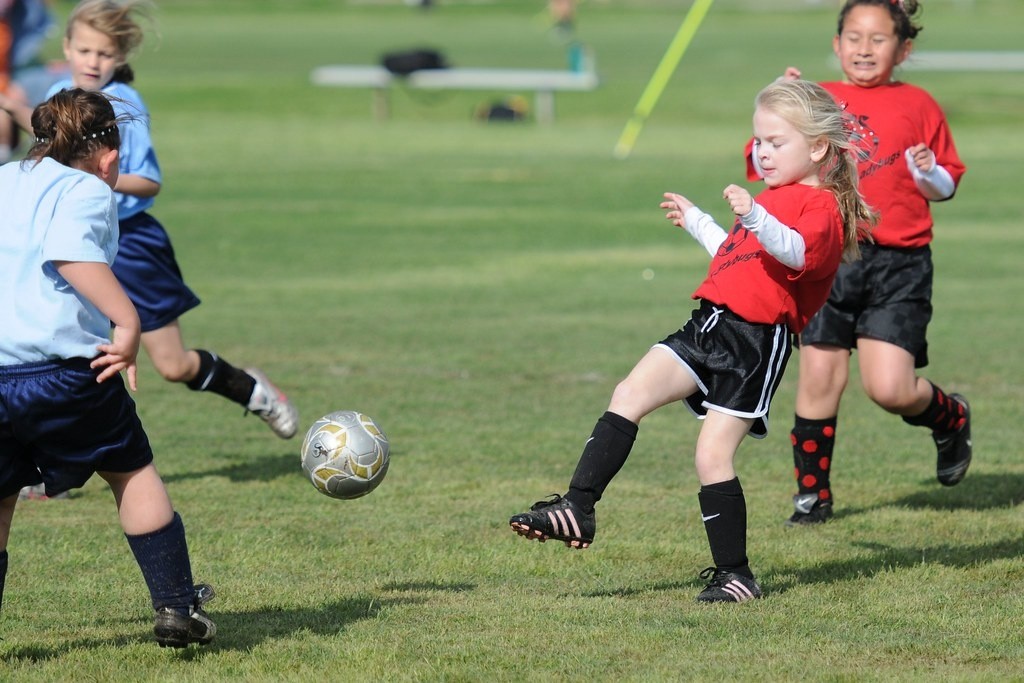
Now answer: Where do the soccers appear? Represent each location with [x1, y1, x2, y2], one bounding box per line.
[300, 409, 391, 500]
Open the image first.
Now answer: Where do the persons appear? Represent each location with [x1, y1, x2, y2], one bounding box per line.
[745, 0, 972, 524]
[0, 0, 297, 646]
[509, 78, 877, 604]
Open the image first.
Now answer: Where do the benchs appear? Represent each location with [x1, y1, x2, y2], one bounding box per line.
[308, 62, 601, 127]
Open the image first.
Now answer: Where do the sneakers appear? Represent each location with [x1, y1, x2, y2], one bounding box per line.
[783, 494, 835, 527]
[17, 480, 69, 499]
[243, 366, 298, 439]
[154, 583, 217, 646]
[695, 567, 760, 603]
[931, 392, 972, 486]
[510, 493, 596, 549]
